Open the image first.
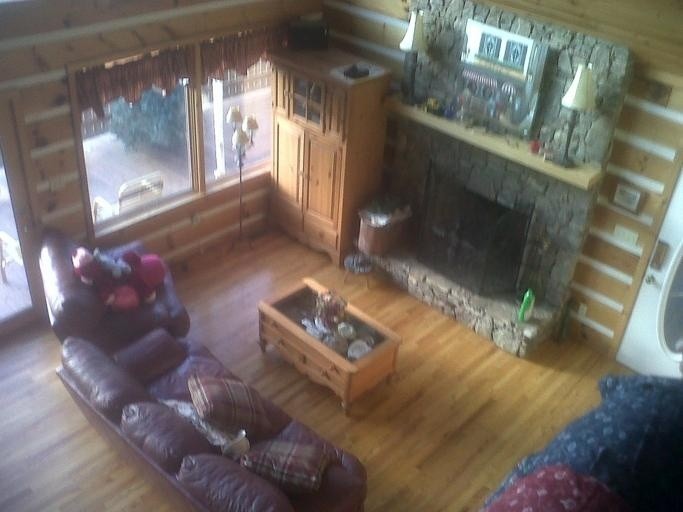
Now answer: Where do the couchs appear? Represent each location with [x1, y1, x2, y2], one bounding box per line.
[55, 326, 366, 512]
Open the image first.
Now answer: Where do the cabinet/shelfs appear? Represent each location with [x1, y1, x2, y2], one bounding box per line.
[270, 47, 391, 268]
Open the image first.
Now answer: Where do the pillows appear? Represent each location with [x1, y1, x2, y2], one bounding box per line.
[186, 373, 336, 496]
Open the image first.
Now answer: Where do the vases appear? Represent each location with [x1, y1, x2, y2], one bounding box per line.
[313, 316, 333, 334]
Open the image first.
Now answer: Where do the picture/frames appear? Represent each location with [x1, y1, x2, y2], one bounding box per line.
[606, 177, 647, 217]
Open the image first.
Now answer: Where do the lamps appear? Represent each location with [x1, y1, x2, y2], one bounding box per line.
[398, 8, 424, 105]
[225, 104, 257, 251]
[558, 60, 596, 166]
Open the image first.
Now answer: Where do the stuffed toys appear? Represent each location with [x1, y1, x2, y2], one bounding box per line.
[69, 243, 168, 312]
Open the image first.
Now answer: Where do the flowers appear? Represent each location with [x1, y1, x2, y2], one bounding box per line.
[314, 288, 345, 329]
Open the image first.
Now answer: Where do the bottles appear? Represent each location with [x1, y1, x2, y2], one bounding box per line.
[519, 288, 536, 322]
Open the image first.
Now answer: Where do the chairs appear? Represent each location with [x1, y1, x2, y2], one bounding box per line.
[90, 171, 164, 225]
[36, 227, 190, 350]
